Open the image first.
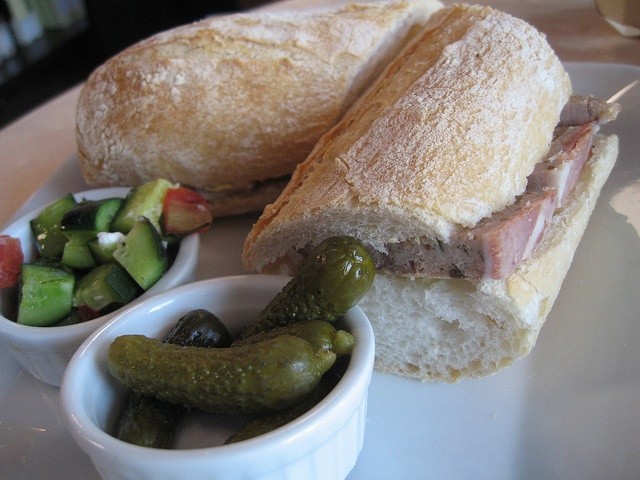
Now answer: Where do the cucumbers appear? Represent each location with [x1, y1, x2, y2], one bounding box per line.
[107, 237, 374, 447]
[19, 179, 177, 327]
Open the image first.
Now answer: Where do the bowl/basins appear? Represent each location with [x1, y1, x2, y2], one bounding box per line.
[0, 181, 202, 387]
[57, 270, 377, 479]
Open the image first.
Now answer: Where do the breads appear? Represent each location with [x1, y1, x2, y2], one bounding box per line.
[77, 2, 445, 217]
[241, 2, 619, 386]
